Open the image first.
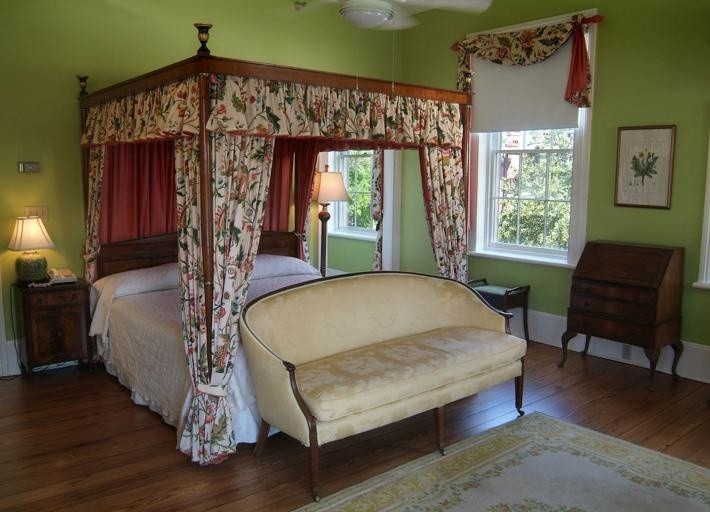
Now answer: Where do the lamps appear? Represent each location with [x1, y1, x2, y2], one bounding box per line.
[337, 0, 396, 32]
[311, 164, 350, 277]
[5, 214, 56, 285]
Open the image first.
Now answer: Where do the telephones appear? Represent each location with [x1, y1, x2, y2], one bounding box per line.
[46, 267, 77, 284]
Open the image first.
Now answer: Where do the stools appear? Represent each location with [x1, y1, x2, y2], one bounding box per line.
[467, 277, 532, 345]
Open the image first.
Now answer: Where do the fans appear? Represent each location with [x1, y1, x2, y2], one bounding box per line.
[331, 1, 494, 31]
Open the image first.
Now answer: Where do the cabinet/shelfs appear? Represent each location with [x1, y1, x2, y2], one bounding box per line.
[556, 239, 686, 392]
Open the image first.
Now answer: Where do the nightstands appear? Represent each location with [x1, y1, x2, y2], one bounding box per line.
[12, 274, 96, 374]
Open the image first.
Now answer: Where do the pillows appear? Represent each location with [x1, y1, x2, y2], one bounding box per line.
[92, 265, 182, 300]
[247, 251, 321, 282]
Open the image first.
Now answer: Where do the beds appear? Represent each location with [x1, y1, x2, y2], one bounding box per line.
[72, 227, 477, 468]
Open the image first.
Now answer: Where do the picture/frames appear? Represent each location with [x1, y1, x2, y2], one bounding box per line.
[613, 125, 678, 211]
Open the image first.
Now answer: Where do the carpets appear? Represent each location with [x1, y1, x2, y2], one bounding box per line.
[286, 407, 710, 512]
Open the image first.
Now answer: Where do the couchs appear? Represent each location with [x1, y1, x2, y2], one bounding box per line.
[237, 267, 528, 503]
